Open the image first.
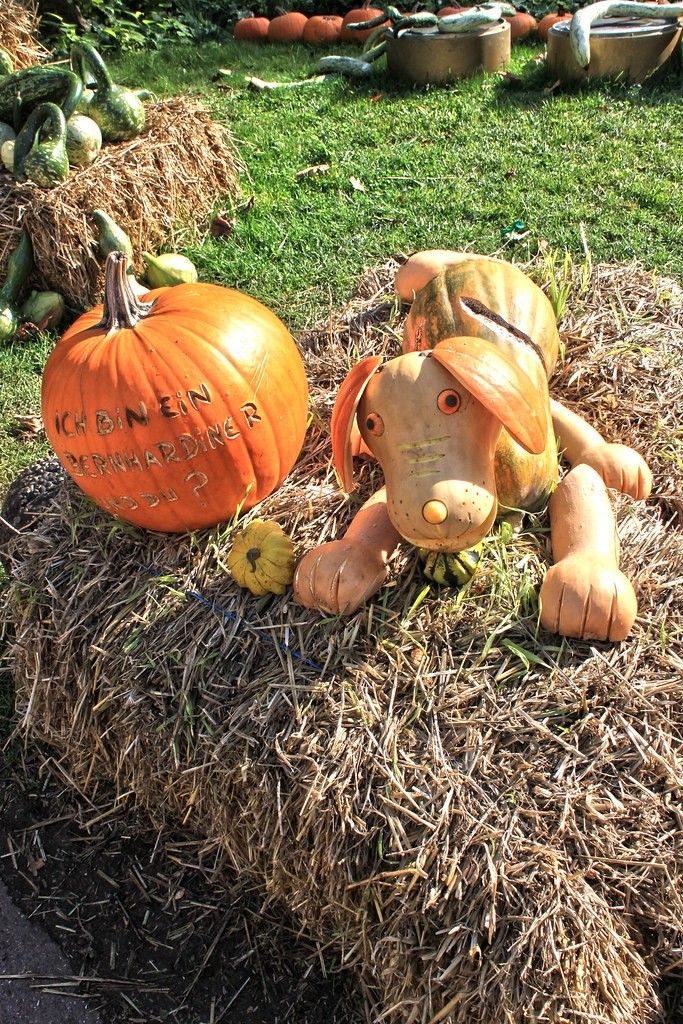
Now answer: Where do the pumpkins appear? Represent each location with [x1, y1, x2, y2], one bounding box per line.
[0, 0, 682, 600]
